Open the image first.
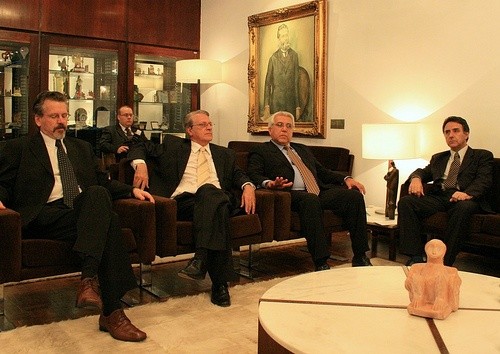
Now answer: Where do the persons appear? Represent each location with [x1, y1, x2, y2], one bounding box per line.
[398, 116, 494, 267]
[127, 110, 256, 307]
[247, 112, 373, 273]
[100, 106, 151, 156]
[94, 107, 108, 127]
[0, 91, 154, 342]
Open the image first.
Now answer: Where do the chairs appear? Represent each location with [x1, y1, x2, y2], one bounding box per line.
[0, 141, 355, 334]
[396, 158, 500, 270]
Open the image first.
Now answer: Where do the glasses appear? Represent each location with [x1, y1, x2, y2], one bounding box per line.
[189, 122, 215, 128]
[119, 113, 133, 118]
[40, 112, 70, 121]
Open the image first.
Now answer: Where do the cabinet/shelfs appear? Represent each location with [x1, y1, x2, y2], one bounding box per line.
[41, 36, 119, 129]
[131, 55, 176, 130]
[0, 29, 33, 140]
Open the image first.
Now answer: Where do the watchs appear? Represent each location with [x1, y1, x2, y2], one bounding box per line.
[344, 175, 352, 180]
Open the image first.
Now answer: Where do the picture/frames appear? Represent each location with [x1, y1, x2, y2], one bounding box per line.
[247, 0, 327, 139]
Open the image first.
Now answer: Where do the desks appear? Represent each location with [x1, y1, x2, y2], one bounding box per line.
[366, 207, 399, 261]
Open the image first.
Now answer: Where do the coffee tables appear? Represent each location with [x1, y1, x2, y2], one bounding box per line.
[257, 266, 500, 354]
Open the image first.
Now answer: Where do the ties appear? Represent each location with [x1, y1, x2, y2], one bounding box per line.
[284, 146, 320, 196]
[55, 138, 79, 209]
[196, 147, 211, 188]
[125, 127, 132, 140]
[443, 152, 460, 192]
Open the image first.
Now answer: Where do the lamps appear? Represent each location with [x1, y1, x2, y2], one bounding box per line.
[175, 59, 223, 111]
[362, 123, 427, 216]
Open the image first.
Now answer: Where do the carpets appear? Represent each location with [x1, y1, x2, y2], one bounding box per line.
[0, 257, 408, 354]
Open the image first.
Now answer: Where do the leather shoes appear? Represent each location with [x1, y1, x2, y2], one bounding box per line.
[75, 275, 103, 311]
[352, 255, 372, 266]
[315, 263, 330, 271]
[98, 308, 147, 342]
[405, 255, 427, 266]
[210, 282, 231, 307]
[177, 257, 206, 281]
